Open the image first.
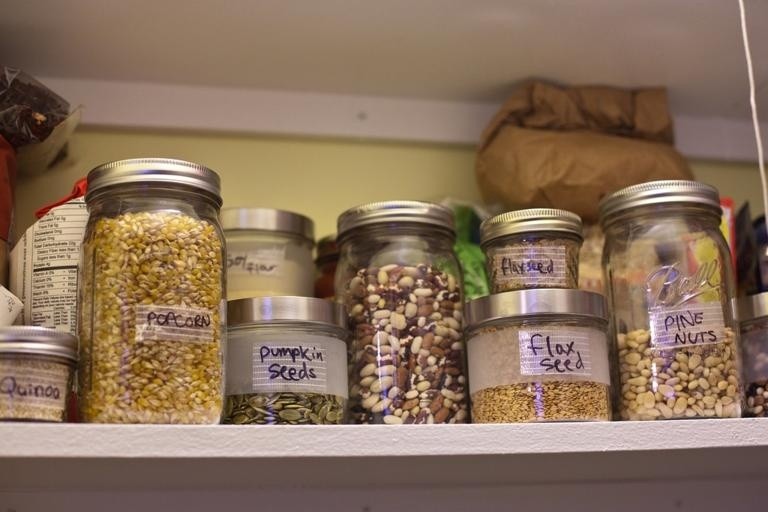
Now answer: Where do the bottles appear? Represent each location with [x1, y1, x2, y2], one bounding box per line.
[311, 234, 338, 298]
[737, 292, 768, 417]
[7, 178, 90, 332]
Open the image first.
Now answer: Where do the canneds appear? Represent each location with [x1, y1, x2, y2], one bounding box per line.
[315, 234, 342, 300]
[730, 289, 767, 416]
